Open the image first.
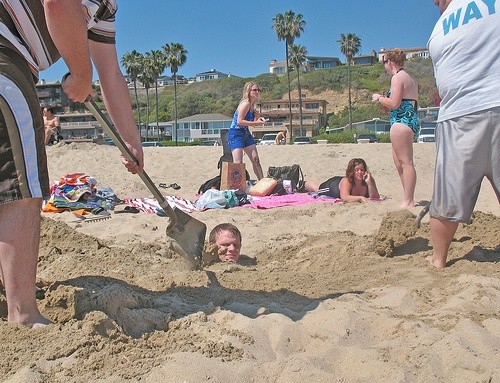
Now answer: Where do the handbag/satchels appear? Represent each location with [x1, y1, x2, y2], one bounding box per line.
[267, 164, 304, 192]
[249, 177, 277, 197]
[220, 162, 246, 195]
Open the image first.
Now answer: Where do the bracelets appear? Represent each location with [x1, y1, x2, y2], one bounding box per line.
[378, 95, 385, 103]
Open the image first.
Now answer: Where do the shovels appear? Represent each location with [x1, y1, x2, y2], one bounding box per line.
[375, 200, 431, 256]
[62, 73, 206, 269]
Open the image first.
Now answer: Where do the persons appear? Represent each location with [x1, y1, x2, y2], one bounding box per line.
[371, 48, 420, 209]
[424, 0, 499, 271]
[297, 157, 380, 204]
[0, 0, 144, 329]
[229, 81, 265, 180]
[40, 106, 61, 146]
[209, 222, 243, 263]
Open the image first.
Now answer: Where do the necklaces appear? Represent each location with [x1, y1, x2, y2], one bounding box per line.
[396, 67, 404, 73]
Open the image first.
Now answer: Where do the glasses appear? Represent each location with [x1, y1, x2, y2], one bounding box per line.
[250, 89, 259, 92]
[383, 60, 388, 64]
